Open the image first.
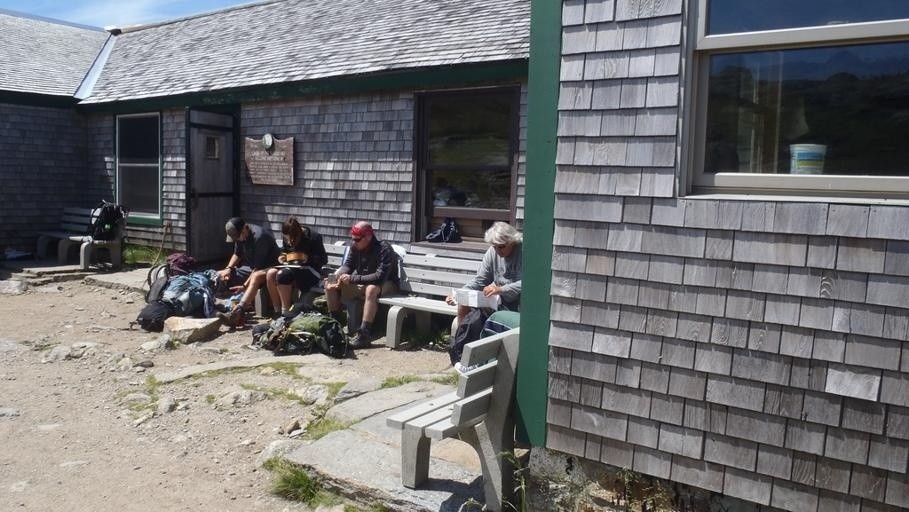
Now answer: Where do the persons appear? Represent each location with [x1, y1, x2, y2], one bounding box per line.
[266, 216, 328, 323]
[329, 222, 399, 346]
[446, 221, 522, 356]
[214, 215, 280, 331]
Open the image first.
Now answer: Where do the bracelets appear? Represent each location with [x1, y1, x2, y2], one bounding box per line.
[225, 267, 233, 269]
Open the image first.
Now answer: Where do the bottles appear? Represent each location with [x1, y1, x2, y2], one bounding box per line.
[326, 273, 342, 314]
[103, 221, 111, 238]
[433, 192, 446, 207]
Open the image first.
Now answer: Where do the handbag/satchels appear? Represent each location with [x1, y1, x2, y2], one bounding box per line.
[93, 205, 113, 240]
[283, 332, 319, 355]
[425, 217, 460, 242]
[446, 308, 495, 367]
[393, 255, 412, 293]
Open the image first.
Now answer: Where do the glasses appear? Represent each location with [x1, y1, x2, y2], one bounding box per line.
[353, 235, 363, 242]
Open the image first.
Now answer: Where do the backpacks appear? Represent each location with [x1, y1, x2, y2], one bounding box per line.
[319, 319, 348, 358]
[138, 301, 169, 332]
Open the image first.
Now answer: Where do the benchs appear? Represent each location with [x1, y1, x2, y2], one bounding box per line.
[382, 325, 522, 511]
[253, 237, 525, 350]
[34, 205, 128, 269]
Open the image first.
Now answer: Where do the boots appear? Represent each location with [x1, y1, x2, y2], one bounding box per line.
[216, 304, 245, 328]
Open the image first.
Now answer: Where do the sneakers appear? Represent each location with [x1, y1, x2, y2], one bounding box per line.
[349, 328, 369, 346]
[454, 361, 486, 374]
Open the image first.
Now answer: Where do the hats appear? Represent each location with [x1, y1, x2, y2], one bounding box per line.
[391, 244, 406, 259]
[226, 217, 245, 242]
[283, 302, 313, 318]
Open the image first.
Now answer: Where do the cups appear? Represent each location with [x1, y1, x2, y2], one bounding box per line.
[232, 299, 245, 327]
